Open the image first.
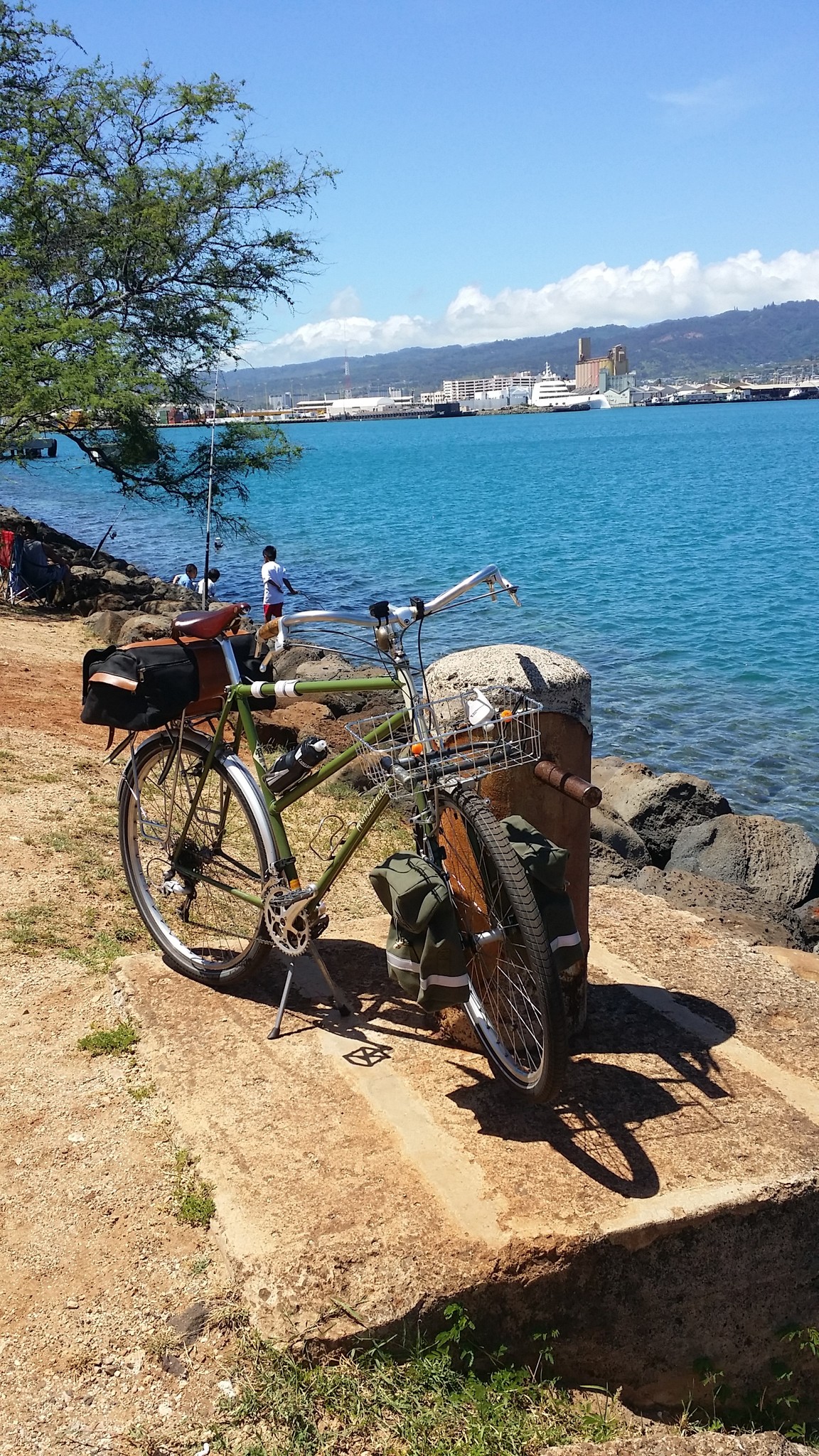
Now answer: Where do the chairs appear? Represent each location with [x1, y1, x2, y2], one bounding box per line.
[0, 529, 74, 611]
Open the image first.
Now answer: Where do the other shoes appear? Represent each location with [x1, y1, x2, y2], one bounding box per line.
[43, 603, 55, 608]
[65, 599, 79, 610]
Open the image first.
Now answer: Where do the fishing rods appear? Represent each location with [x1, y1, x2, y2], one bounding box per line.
[90, 450, 173, 561]
[201, 336, 224, 611]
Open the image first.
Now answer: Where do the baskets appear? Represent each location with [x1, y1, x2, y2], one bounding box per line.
[345, 686, 544, 800]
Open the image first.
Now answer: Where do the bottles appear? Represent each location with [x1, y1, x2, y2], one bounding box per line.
[265, 738, 327, 793]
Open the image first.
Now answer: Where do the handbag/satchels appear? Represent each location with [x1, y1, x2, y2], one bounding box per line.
[495, 814, 585, 974]
[80, 629, 277, 731]
[368, 852, 470, 1013]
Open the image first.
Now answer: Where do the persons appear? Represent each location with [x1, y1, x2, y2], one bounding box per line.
[198, 568, 221, 602]
[2, 519, 87, 596]
[261, 545, 296, 624]
[172, 564, 197, 592]
